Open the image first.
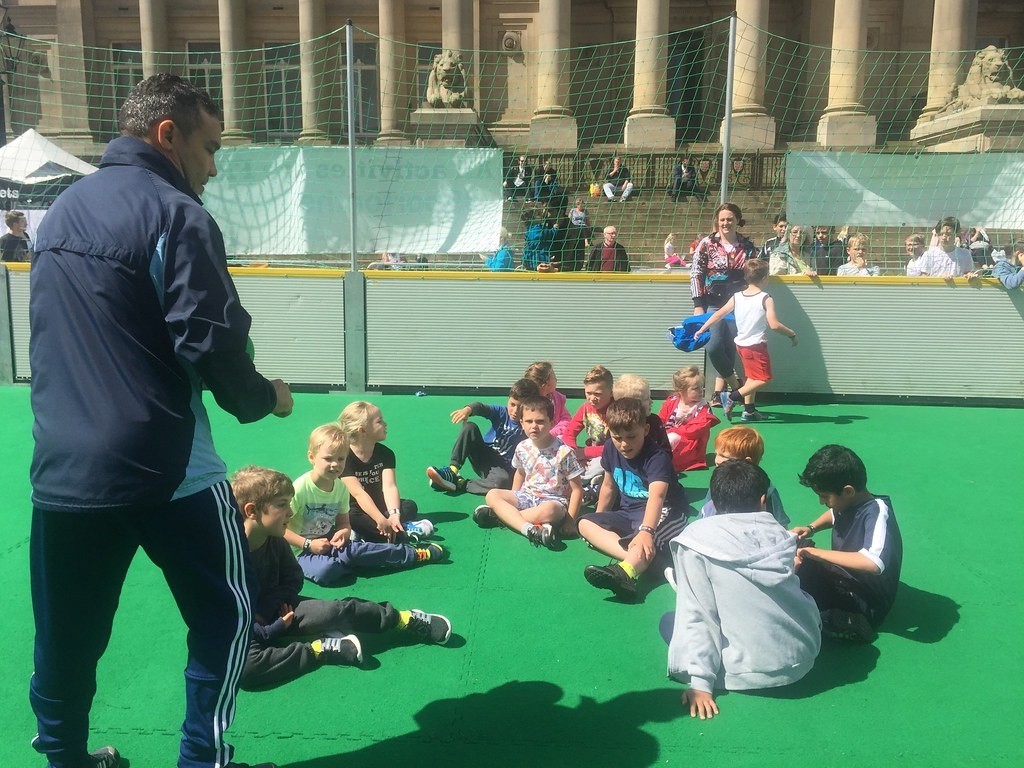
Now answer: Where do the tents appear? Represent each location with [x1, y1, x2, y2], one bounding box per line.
[0, 127, 100, 184]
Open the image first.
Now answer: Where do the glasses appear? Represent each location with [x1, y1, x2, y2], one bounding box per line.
[608, 233, 616, 235]
[790, 230, 805, 236]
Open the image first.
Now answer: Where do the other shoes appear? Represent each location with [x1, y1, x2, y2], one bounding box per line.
[664, 567, 678, 592]
[591, 475, 603, 485]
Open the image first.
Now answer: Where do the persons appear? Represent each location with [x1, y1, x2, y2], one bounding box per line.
[788, 444, 904, 644]
[338, 400, 435, 542]
[473, 395, 582, 551]
[695, 425, 795, 528]
[356, 153, 1024, 289]
[284, 423, 446, 586]
[693, 258, 798, 422]
[230, 464, 455, 691]
[574, 396, 688, 604]
[26, 73, 294, 768]
[425, 361, 721, 493]
[690, 203, 758, 407]
[659, 457, 823, 720]
[0, 209, 33, 261]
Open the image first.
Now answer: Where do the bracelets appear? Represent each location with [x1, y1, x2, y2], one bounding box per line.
[388, 508, 400, 515]
[638, 525, 657, 536]
[806, 525, 815, 533]
[303, 538, 312, 550]
[788, 333, 796, 338]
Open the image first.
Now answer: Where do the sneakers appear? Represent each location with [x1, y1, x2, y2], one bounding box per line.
[720, 392, 734, 423]
[416, 543, 443, 563]
[90, 746, 119, 768]
[739, 401, 757, 406]
[711, 391, 721, 406]
[473, 506, 507, 528]
[318, 631, 363, 664]
[742, 410, 768, 421]
[430, 473, 461, 490]
[404, 609, 451, 645]
[584, 565, 638, 598]
[403, 520, 434, 541]
[527, 523, 560, 550]
[428, 467, 457, 491]
[226, 762, 250, 768]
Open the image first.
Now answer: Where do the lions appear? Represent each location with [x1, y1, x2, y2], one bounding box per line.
[934, 44, 1024, 119]
[426, 49, 468, 108]
